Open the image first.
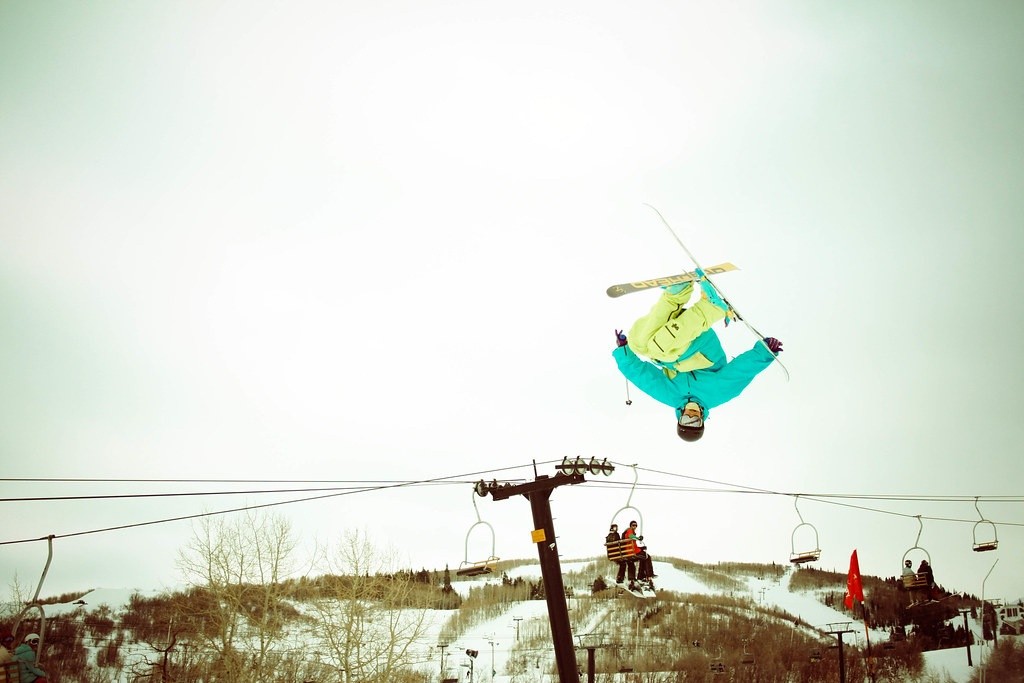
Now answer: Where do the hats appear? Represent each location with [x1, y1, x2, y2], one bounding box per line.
[921, 560, 929, 565]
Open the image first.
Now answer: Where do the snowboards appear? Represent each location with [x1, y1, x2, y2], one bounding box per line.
[604, 576, 657, 598]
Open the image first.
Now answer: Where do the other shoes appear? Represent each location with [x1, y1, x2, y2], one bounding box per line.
[616, 579, 625, 584]
[647, 574, 659, 581]
[628, 576, 637, 581]
[637, 575, 647, 580]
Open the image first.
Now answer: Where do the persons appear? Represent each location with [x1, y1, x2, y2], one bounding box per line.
[904, 560, 934, 607]
[613, 266, 784, 443]
[606, 521, 655, 590]
[0, 634, 50, 683]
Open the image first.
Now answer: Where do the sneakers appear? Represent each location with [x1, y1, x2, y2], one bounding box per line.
[701, 280, 735, 328]
[662, 280, 695, 296]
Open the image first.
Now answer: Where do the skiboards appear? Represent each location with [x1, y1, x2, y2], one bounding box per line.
[605, 205, 791, 381]
[906, 590, 962, 610]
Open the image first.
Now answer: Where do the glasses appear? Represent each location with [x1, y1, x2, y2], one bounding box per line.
[632, 525, 637, 527]
[679, 414, 703, 428]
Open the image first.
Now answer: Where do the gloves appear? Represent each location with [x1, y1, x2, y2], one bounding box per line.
[615, 329, 628, 348]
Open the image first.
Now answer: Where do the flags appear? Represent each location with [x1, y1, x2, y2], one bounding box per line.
[843, 550, 864, 610]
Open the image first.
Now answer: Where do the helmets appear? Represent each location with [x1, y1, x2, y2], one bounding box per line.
[677, 415, 705, 442]
[904, 560, 912, 566]
